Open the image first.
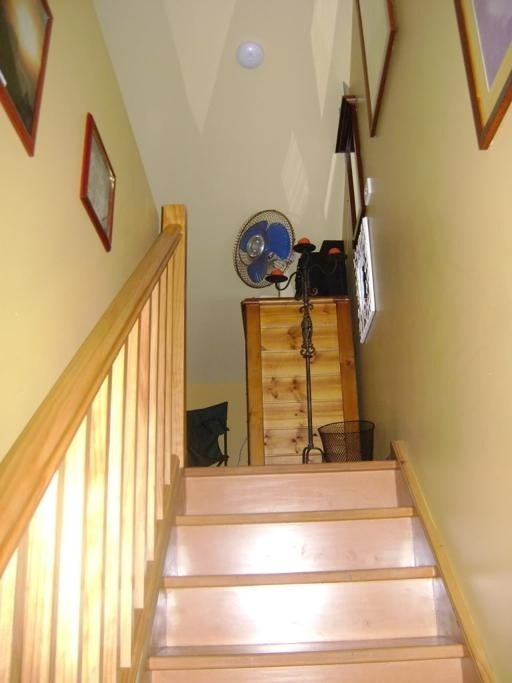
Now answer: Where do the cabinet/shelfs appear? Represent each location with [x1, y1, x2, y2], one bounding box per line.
[240, 297, 363, 466]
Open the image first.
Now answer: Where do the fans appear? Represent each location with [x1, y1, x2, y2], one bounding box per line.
[231, 208, 296, 298]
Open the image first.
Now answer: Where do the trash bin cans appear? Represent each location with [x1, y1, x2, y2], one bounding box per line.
[317, 420, 375, 462]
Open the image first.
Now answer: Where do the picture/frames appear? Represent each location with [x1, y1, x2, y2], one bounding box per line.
[0, 0, 54, 158]
[78, 112, 117, 253]
[452, 0, 512, 153]
